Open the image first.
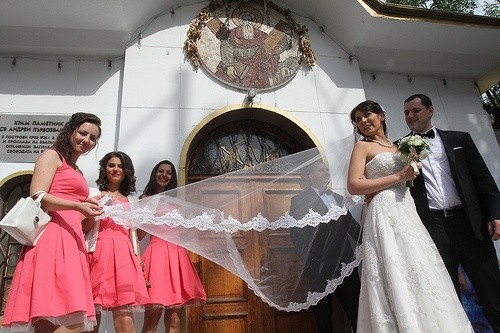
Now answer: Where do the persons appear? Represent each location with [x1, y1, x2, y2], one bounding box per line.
[86, 151, 151, 333]
[347, 99, 476, 332]
[136, 160, 208, 333]
[363, 93, 500, 332]
[290, 161, 364, 333]
[0, 111, 106, 333]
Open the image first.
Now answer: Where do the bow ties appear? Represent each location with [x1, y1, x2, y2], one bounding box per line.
[413, 129, 435, 138]
[318, 189, 332, 196]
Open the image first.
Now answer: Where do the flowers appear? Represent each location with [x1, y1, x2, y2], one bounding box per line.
[397, 135, 432, 187]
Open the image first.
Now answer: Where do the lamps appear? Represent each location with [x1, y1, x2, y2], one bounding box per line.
[247, 89, 257, 101]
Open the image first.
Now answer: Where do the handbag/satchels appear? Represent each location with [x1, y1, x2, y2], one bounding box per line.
[0, 191, 52, 247]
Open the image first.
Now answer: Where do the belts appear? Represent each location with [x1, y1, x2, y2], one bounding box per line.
[430, 206, 464, 218]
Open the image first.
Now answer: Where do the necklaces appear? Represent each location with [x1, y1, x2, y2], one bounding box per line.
[368, 137, 394, 148]
